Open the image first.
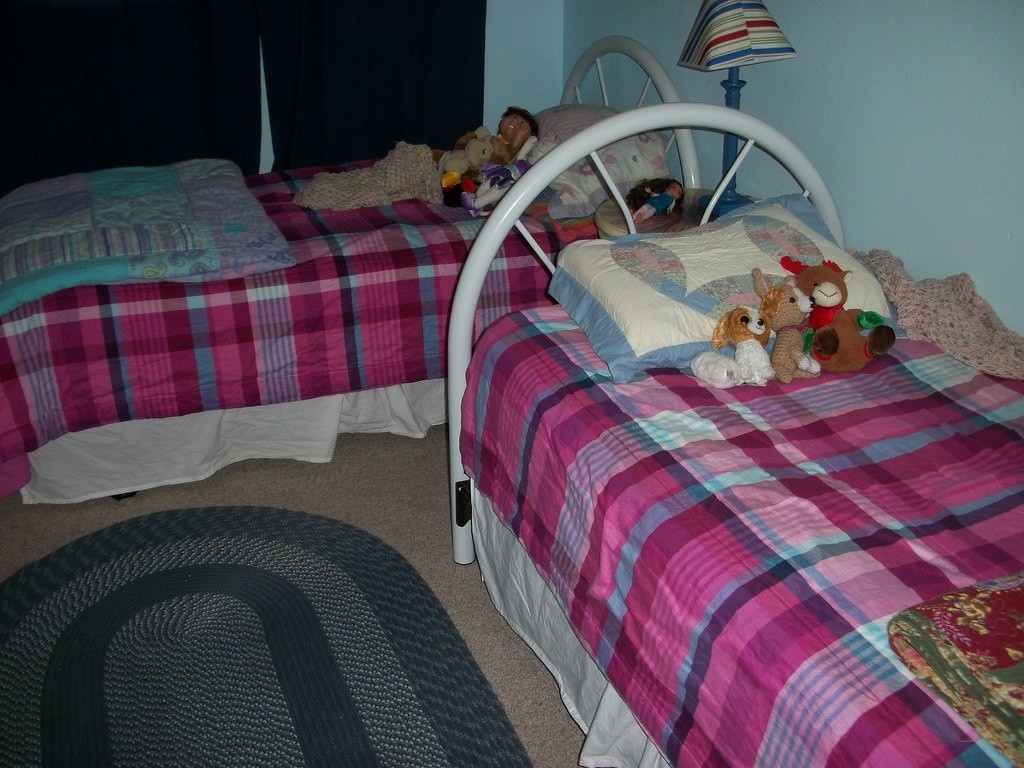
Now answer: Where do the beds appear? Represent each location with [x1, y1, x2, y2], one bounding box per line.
[0, 34, 698, 505]
[445, 102, 1024, 768]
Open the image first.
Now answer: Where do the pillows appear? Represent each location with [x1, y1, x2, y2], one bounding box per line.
[548, 190, 905, 384]
[533, 104, 672, 219]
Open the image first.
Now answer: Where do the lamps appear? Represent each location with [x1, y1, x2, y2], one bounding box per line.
[676, 0, 797, 222]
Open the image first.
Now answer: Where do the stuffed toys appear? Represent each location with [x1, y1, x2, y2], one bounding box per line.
[690, 255, 896, 389]
[431, 106, 539, 187]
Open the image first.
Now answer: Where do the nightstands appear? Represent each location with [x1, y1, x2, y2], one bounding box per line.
[594, 189, 761, 240]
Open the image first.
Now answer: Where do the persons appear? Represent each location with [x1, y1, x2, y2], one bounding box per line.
[627, 177, 685, 224]
[458, 136, 565, 218]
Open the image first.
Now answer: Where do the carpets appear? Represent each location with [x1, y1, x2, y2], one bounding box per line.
[0, 506, 533, 768]
